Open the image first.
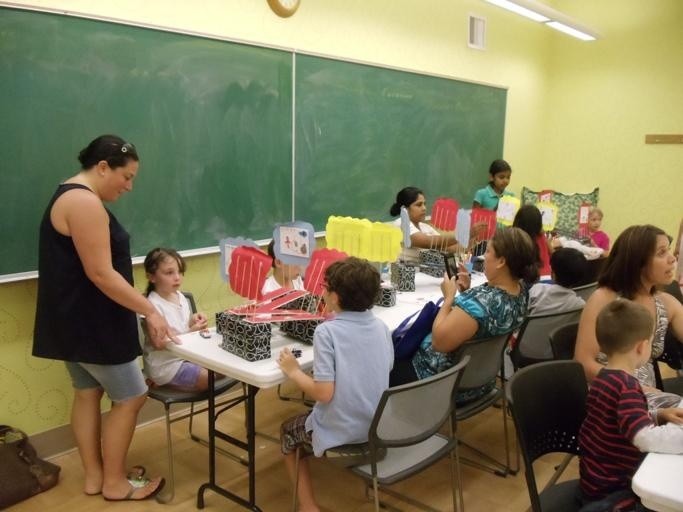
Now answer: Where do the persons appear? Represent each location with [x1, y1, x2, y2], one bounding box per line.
[382, 227, 541, 408]
[580, 209, 612, 259]
[509, 207, 552, 279]
[27, 133, 184, 500]
[469, 158, 517, 215]
[567, 301, 681, 511]
[274, 258, 396, 510]
[570, 223, 681, 426]
[512, 242, 589, 367]
[385, 185, 491, 256]
[138, 246, 232, 392]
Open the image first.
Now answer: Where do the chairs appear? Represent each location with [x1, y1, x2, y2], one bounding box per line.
[434, 319, 512, 511]
[550, 321, 665, 425]
[509, 360, 616, 510]
[127, 291, 255, 505]
[289, 354, 471, 512]
[509, 299, 585, 477]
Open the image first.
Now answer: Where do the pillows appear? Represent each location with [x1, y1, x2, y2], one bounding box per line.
[520, 188, 603, 237]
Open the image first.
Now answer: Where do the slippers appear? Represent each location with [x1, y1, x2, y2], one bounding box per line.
[103, 475, 166, 501]
[125, 463, 146, 482]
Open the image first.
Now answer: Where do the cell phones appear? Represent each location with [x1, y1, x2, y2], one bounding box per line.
[444, 253, 458, 280]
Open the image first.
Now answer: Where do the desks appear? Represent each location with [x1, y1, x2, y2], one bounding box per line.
[630, 450, 683, 511]
[168, 251, 551, 511]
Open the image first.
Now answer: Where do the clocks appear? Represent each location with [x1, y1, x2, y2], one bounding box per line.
[266, 0, 304, 21]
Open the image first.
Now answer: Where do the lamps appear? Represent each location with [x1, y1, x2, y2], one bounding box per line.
[477, 0, 605, 44]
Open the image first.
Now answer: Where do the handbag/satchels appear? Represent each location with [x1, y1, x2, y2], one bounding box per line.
[0, 423, 61, 512]
[391, 297, 444, 361]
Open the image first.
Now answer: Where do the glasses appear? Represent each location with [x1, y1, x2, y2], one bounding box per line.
[122, 141, 135, 152]
